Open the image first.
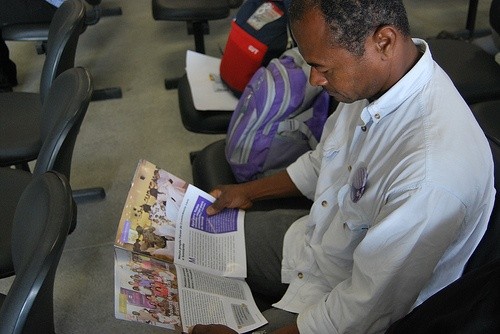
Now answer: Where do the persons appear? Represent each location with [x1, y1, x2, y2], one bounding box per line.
[0, 0, 102, 93]
[124, 169, 186, 328]
[188, 0, 496, 334]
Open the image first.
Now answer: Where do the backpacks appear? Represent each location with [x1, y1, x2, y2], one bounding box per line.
[218, 0, 288, 98]
[224, 46, 331, 182]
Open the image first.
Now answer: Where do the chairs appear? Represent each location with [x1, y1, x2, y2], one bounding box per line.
[0, 170, 76, 334]
[152, 0, 244, 89]
[0, 0, 122, 103]
[177, 74, 234, 165]
[419, 0, 500, 191]
[0, 0, 105, 204]
[193, 138, 322, 209]
